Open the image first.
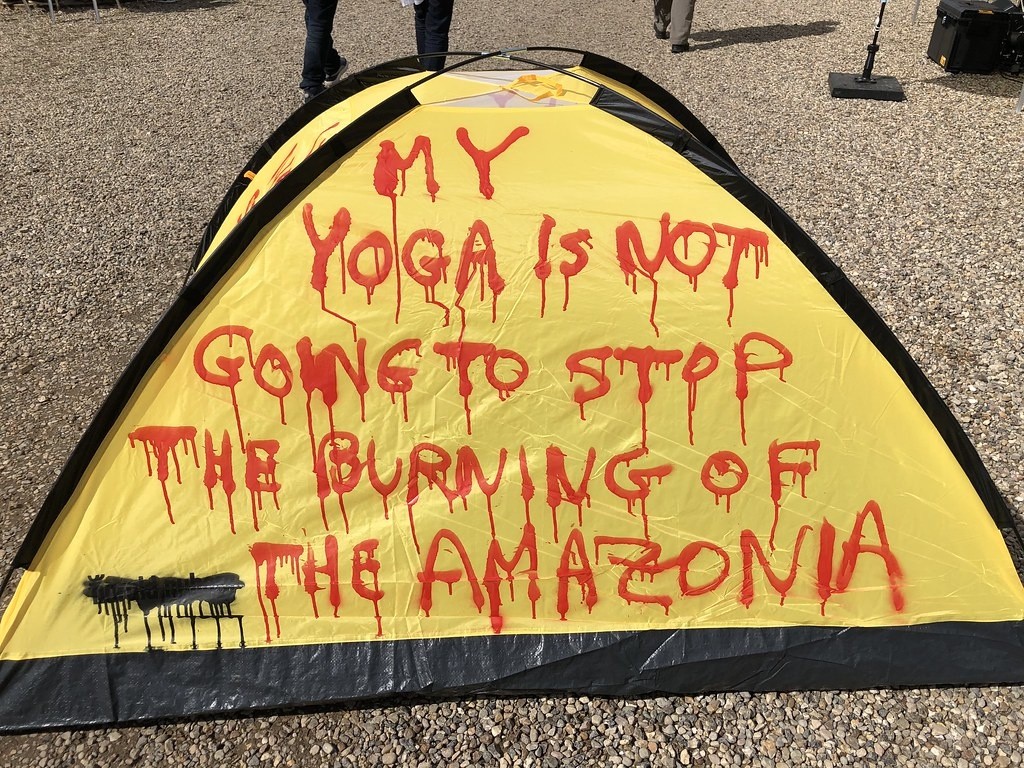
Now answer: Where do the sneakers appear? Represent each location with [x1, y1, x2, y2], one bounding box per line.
[656, 31, 667, 39]
[324, 57, 349, 86]
[303, 88, 326, 106]
[671, 43, 690, 52]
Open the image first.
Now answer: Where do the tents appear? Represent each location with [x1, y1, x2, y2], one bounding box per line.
[0, 44, 1021, 734]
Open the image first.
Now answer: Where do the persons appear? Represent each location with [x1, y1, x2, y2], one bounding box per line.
[299, 1, 348, 103]
[652, 0, 696, 52]
[400, 0, 455, 71]
